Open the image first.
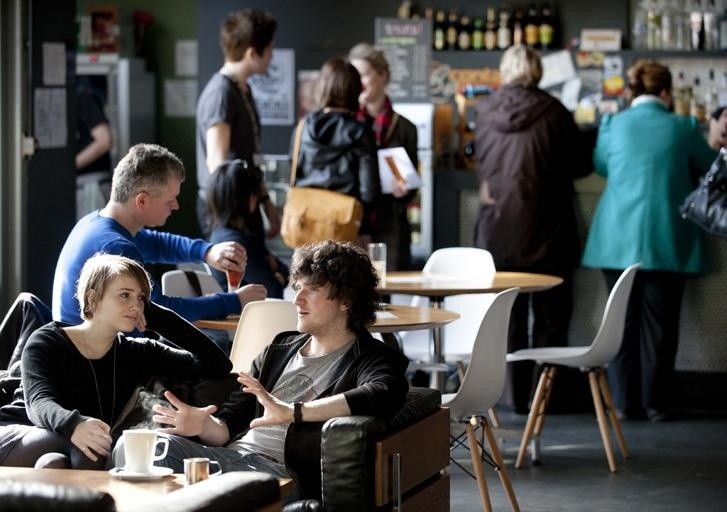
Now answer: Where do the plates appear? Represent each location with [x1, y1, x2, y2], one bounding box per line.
[108, 466, 174, 480]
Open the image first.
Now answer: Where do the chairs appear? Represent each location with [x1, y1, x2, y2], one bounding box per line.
[1, 469, 282, 511]
[506, 261, 642, 473]
[159, 268, 223, 298]
[437, 286, 522, 511]
[229, 298, 298, 375]
[397, 244, 498, 428]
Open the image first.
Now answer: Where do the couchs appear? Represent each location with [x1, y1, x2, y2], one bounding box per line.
[1, 375, 451, 511]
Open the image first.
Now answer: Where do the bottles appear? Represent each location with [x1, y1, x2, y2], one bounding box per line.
[397, 0, 556, 53]
[225, 259, 246, 294]
[630, 0, 727, 124]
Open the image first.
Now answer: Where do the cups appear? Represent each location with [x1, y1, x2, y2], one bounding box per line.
[122, 429, 170, 473]
[183, 457, 223, 486]
[367, 242, 387, 289]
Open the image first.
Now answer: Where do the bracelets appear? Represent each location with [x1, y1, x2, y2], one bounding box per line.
[294, 401, 303, 426]
[260, 194, 269, 204]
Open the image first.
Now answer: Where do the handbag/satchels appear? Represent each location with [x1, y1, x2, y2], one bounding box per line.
[679, 146, 727, 237]
[280, 186, 364, 250]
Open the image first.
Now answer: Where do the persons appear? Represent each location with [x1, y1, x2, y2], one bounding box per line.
[194, 8, 280, 242]
[151, 238, 410, 506]
[287, 57, 387, 242]
[344, 43, 421, 272]
[475, 43, 594, 414]
[72, 91, 114, 220]
[580, 62, 720, 423]
[206, 159, 283, 300]
[706, 106, 727, 151]
[51, 143, 268, 326]
[0, 254, 231, 470]
